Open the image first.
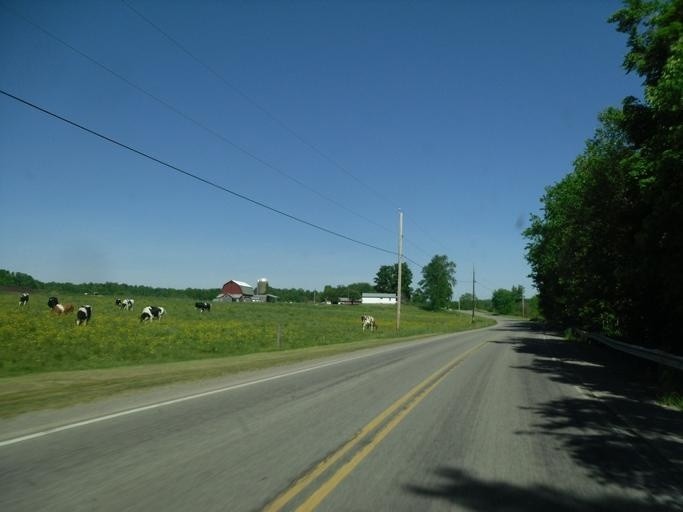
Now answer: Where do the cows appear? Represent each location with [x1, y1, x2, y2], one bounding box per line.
[17, 293, 29, 306]
[77, 304, 93, 327]
[48, 297, 74, 317]
[116, 299, 134, 313]
[361, 315, 378, 333]
[195, 302, 210, 313]
[141, 306, 166, 321]
